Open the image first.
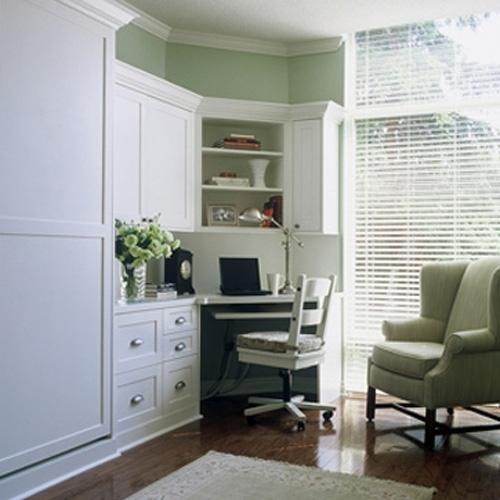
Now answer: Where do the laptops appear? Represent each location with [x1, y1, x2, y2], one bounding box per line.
[218, 256, 273, 295]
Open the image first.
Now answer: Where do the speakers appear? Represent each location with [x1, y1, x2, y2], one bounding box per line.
[164, 246, 196, 295]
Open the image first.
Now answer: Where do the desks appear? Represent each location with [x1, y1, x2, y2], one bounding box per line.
[198, 288, 342, 404]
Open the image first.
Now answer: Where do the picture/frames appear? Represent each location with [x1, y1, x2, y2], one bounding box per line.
[206, 202, 241, 226]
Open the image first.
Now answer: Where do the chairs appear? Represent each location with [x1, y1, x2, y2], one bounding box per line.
[236, 273, 337, 430]
[367, 260, 500, 458]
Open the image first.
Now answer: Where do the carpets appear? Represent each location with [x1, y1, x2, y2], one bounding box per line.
[125, 450, 436, 500]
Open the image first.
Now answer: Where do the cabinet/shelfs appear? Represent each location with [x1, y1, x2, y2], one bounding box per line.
[115, 300, 203, 454]
[114, 59, 202, 234]
[201, 96, 289, 234]
[289, 100, 344, 236]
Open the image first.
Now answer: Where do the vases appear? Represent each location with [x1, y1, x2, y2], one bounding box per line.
[247, 159, 270, 188]
[119, 264, 146, 304]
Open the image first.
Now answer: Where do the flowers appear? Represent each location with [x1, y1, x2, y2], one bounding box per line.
[114, 210, 181, 297]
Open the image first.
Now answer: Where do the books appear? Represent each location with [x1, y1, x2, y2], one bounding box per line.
[146, 282, 177, 300]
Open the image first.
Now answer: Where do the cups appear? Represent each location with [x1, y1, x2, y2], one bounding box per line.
[266, 274, 286, 296]
